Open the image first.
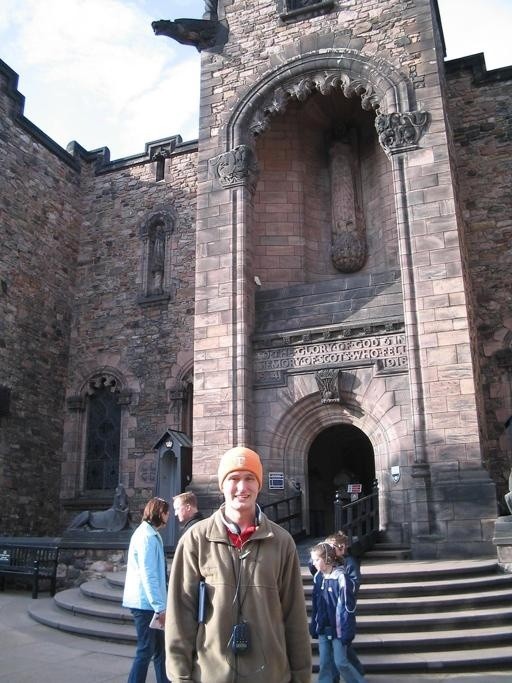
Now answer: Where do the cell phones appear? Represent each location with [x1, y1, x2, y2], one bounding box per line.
[231, 624, 249, 654]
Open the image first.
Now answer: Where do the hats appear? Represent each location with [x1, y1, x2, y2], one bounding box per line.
[218, 446, 263, 488]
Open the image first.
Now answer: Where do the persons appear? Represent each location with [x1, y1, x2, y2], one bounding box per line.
[121, 496, 172, 683]
[172, 490, 203, 533]
[164, 446, 313, 683]
[309, 543, 365, 683]
[307, 532, 366, 675]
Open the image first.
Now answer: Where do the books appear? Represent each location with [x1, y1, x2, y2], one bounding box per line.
[148, 613, 166, 632]
[197, 581, 206, 625]
